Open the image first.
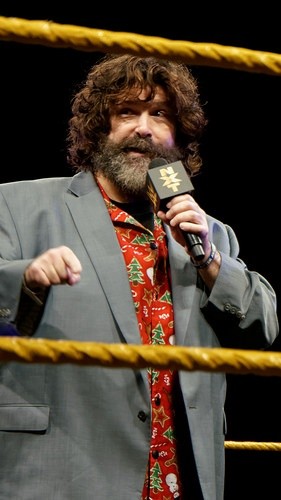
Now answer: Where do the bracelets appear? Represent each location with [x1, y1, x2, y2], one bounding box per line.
[190, 240, 216, 269]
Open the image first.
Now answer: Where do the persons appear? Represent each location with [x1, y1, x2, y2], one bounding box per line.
[0, 52, 281, 500]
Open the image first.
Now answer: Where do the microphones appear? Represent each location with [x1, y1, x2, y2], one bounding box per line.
[145, 158, 205, 260]
